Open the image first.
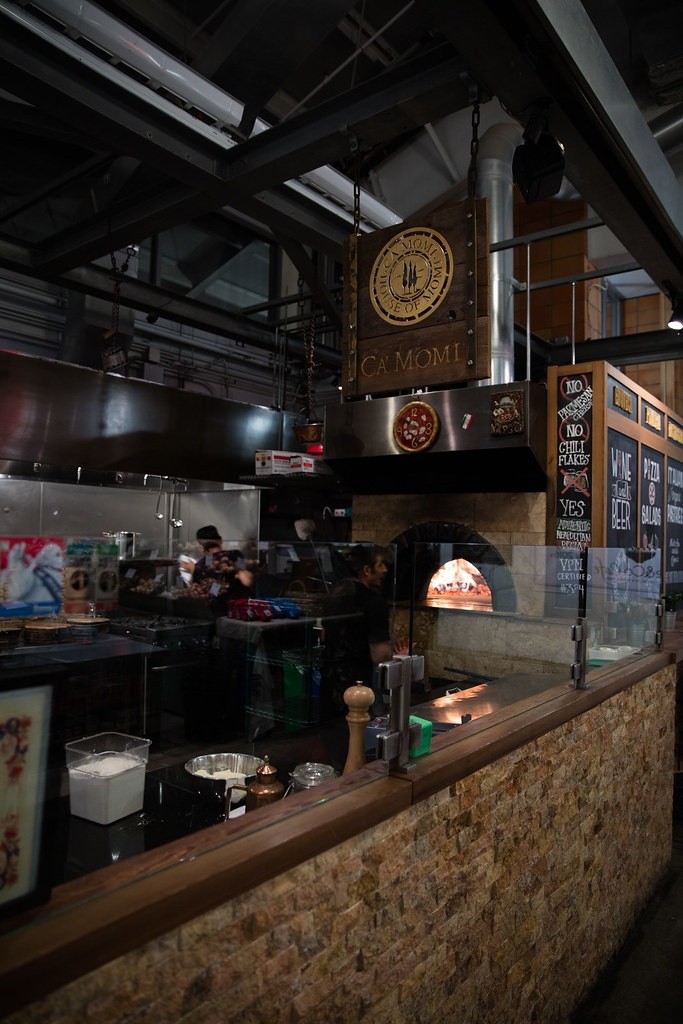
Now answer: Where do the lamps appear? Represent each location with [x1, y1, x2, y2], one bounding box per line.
[100, 247, 136, 374]
[667, 302, 683, 331]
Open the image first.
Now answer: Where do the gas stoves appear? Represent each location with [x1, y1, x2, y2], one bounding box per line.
[113, 615, 214, 644]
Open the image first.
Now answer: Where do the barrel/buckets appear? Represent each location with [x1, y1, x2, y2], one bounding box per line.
[291, 408, 323, 443]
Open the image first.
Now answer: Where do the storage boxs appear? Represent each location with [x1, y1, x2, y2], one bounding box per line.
[254, 449, 322, 476]
[290, 455, 333, 476]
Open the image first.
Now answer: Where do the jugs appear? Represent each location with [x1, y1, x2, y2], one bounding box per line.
[226, 761, 292, 822]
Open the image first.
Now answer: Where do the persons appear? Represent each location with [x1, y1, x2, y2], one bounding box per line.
[187, 525, 257, 617]
[319, 543, 418, 772]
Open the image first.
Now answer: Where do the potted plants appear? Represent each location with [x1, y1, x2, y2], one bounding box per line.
[650, 592, 683, 628]
[618, 597, 658, 641]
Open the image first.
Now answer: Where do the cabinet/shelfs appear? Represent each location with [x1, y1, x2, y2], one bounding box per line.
[216, 609, 369, 739]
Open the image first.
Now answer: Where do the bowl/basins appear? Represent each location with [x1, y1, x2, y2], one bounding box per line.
[184, 753, 265, 796]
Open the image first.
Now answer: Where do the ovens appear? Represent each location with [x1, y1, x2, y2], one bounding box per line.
[141, 638, 220, 737]
